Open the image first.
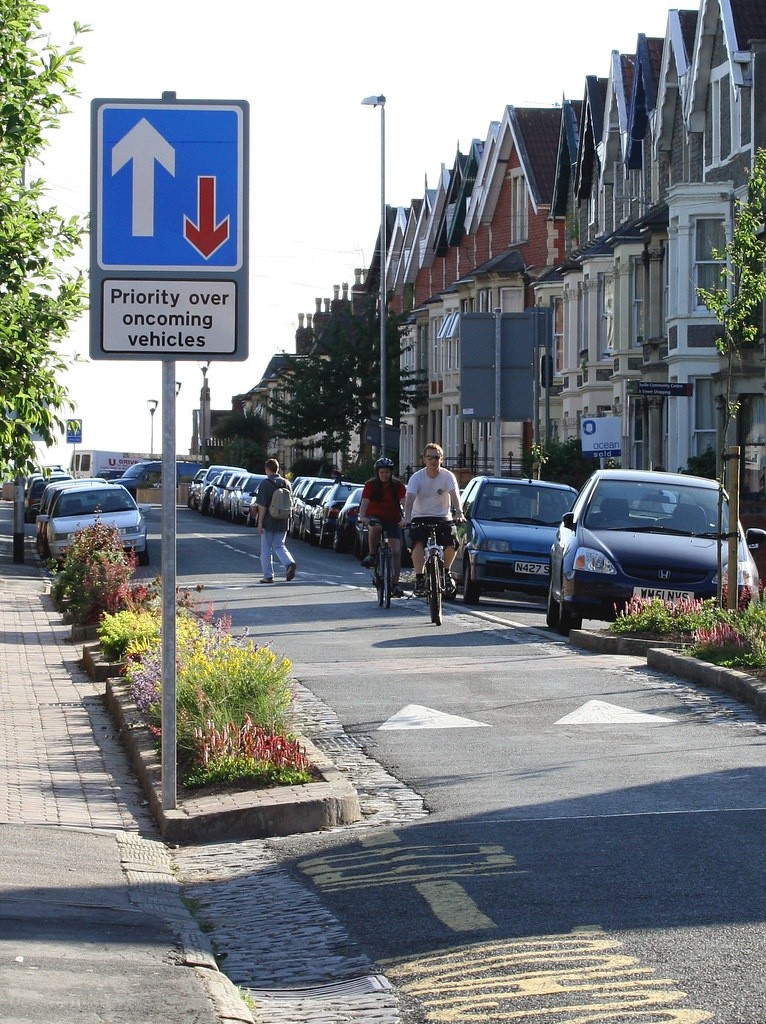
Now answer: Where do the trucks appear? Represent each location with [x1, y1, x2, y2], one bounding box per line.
[69, 450, 155, 479]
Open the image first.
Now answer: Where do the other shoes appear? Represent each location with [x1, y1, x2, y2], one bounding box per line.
[392, 586, 404, 595]
[415, 579, 425, 592]
[363, 556, 376, 564]
[442, 572, 454, 586]
[286, 562, 296, 581]
[260, 578, 273, 582]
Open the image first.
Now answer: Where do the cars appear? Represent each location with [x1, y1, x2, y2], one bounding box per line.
[443, 475, 579, 605]
[35, 483, 149, 569]
[546, 468, 766, 636]
[290, 476, 365, 554]
[25, 464, 110, 558]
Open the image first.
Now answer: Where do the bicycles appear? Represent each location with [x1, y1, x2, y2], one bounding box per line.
[357, 520, 399, 608]
[400, 520, 463, 626]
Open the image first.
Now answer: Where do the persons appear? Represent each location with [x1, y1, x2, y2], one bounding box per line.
[255, 458, 296, 583]
[398, 442, 467, 595]
[360, 457, 409, 595]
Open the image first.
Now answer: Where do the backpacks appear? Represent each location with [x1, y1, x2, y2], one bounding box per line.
[265, 479, 293, 520]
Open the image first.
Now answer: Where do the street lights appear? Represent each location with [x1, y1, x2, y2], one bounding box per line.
[361, 96, 386, 458]
[198, 361, 210, 469]
[147, 400, 158, 454]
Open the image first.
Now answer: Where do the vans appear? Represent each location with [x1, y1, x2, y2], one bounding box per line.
[95, 469, 125, 480]
[107, 461, 202, 502]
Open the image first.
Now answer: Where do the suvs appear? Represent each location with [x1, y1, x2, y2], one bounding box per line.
[189, 465, 268, 528]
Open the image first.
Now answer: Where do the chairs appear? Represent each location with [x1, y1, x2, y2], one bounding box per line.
[664, 503, 708, 535]
[589, 496, 633, 527]
[101, 496, 128, 511]
[61, 499, 90, 515]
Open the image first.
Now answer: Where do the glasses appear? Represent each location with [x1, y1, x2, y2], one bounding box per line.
[425, 456, 442, 460]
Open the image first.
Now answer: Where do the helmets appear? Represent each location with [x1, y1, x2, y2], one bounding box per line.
[374, 458, 394, 470]
